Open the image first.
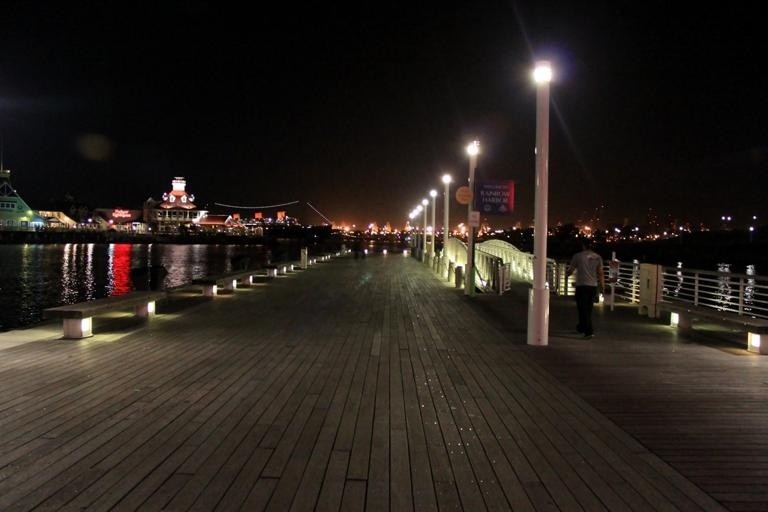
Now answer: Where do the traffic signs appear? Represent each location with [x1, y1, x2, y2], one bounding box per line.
[407, 187, 438, 269]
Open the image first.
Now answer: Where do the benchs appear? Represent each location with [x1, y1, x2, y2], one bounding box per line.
[657, 300, 768, 354]
[41, 263, 297, 340]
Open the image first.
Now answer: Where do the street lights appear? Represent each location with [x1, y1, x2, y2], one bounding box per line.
[524, 59, 551, 348]
[463, 140, 479, 298]
[439, 172, 450, 279]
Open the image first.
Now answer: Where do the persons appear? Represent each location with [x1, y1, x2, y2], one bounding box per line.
[566, 238, 605, 339]
